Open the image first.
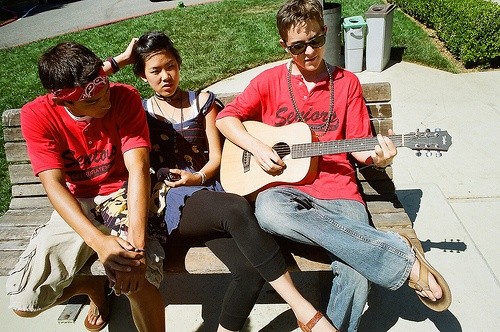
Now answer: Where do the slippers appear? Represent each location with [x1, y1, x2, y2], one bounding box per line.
[84, 285, 116, 332]
[399, 232, 452, 312]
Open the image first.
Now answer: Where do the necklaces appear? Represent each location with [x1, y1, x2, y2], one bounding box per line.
[287, 57, 334, 136]
[152, 87, 184, 137]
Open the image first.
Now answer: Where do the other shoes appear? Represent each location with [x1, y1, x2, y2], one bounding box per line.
[297, 310, 340, 332]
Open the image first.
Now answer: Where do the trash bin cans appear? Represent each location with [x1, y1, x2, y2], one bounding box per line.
[365, 4, 398, 72]
[324, 2, 344, 69]
[342, 16, 365, 73]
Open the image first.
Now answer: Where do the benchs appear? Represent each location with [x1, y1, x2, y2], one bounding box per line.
[0, 81, 426, 323]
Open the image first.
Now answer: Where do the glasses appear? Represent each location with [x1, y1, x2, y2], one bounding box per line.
[282, 27, 327, 55]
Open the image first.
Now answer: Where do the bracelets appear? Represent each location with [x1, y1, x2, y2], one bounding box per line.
[193, 172, 206, 186]
[105, 57, 120, 73]
[130, 248, 145, 253]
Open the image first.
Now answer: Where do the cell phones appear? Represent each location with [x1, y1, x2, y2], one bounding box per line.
[166, 173, 181, 182]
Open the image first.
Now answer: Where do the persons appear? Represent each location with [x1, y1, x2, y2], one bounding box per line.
[215, 0, 452, 332]
[101, 32, 341, 332]
[6, 42, 166, 332]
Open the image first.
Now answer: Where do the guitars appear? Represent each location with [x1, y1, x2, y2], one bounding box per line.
[219, 120, 452, 197]
